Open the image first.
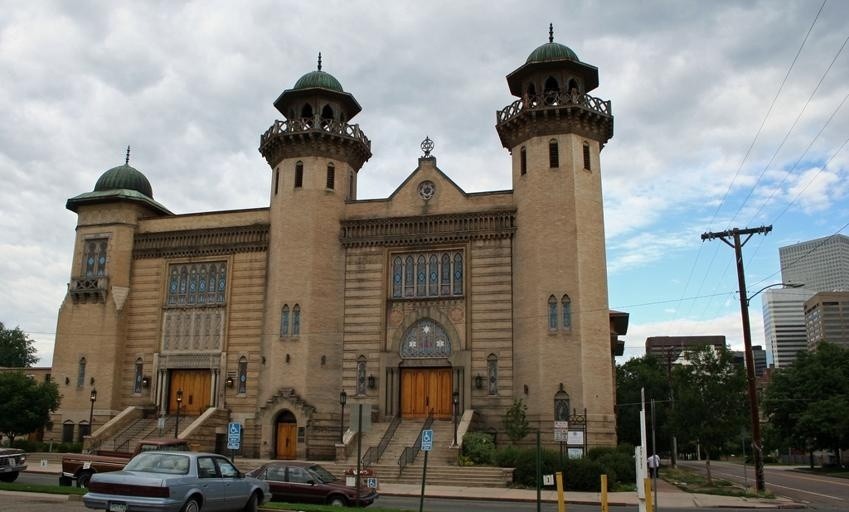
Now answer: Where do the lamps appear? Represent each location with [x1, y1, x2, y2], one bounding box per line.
[368, 374, 375, 387]
[476, 373, 481, 388]
[227, 375, 233, 387]
[142, 377, 150, 388]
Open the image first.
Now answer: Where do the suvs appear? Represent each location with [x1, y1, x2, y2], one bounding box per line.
[0, 447, 27, 481]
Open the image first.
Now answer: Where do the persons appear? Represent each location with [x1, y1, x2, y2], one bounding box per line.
[648, 451, 661, 478]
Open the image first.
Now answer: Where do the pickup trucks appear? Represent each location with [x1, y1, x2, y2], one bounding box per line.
[62, 438, 189, 488]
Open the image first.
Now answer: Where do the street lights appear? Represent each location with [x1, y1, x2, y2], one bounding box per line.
[737, 282, 806, 495]
[340, 388, 347, 442]
[88, 387, 96, 434]
[453, 390, 460, 445]
[176, 387, 184, 438]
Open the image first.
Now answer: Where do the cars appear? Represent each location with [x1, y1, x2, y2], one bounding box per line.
[83, 449, 272, 511]
[246, 460, 378, 507]
